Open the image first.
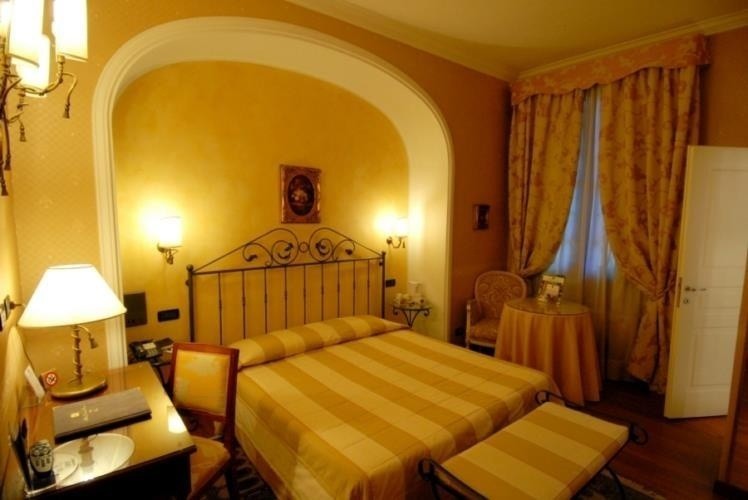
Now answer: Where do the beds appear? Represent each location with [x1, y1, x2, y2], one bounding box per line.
[183, 223, 569, 499]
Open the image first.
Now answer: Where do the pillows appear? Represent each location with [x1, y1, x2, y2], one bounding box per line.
[221, 315, 413, 374]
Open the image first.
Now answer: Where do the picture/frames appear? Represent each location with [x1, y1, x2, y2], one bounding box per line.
[278, 162, 323, 224]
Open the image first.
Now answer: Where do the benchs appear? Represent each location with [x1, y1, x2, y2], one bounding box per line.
[411, 385, 634, 499]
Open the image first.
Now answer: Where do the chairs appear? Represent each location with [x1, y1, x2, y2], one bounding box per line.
[160, 339, 243, 500]
[461, 269, 527, 354]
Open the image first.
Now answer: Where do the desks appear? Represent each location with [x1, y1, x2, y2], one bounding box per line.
[124, 342, 176, 387]
[389, 300, 433, 331]
[495, 296, 604, 407]
[0, 356, 199, 500]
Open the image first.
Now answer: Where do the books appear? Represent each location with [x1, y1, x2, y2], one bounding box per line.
[49, 384, 153, 441]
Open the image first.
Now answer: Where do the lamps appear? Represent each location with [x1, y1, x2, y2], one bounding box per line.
[2, 0, 92, 200]
[15, 262, 130, 403]
[383, 218, 410, 250]
[158, 228, 184, 267]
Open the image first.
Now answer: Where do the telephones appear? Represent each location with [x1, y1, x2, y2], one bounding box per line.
[129, 339, 163, 361]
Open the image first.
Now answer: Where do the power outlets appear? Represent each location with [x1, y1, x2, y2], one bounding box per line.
[4, 293, 13, 321]
